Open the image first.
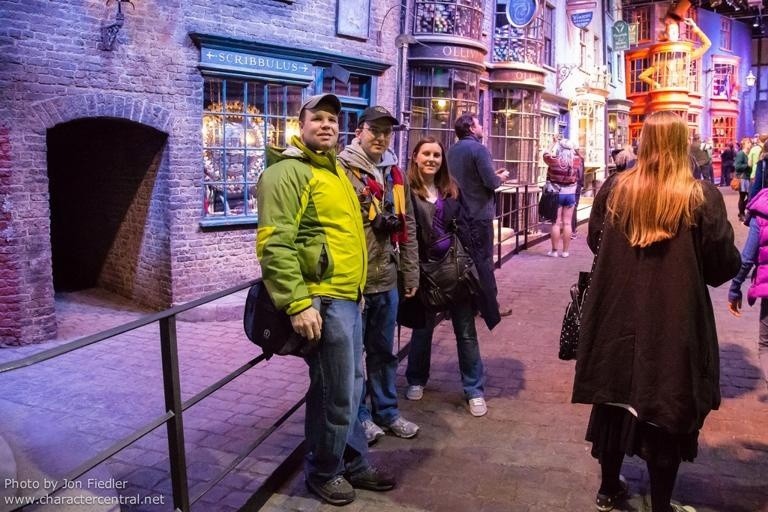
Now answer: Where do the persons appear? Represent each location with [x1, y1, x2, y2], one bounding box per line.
[338, 106, 421, 447]
[397, 136, 502, 417]
[256, 93, 396, 506]
[446, 112, 512, 318]
[541, 111, 768, 512]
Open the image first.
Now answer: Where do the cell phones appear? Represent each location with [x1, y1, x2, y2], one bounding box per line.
[558, 132, 564, 141]
[502, 170, 509, 175]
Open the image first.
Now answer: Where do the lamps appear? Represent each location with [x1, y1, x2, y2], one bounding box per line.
[743, 69, 757, 96]
[378, 4, 416, 49]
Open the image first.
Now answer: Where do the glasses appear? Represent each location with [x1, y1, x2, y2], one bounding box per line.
[362, 126, 393, 138]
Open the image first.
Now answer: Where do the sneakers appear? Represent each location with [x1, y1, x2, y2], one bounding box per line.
[595, 474, 627, 512]
[407, 385, 423, 400]
[547, 251, 569, 257]
[670, 503, 696, 512]
[306, 414, 420, 505]
[469, 398, 488, 417]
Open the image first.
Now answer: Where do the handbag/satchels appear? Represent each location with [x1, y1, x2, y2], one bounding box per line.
[538, 188, 559, 223]
[419, 234, 483, 310]
[558, 281, 590, 361]
[244, 280, 325, 357]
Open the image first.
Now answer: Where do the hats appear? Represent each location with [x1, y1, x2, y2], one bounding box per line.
[299, 93, 341, 117]
[358, 106, 399, 127]
[559, 138, 573, 150]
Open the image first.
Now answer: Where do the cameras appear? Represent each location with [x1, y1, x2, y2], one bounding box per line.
[371, 210, 404, 236]
